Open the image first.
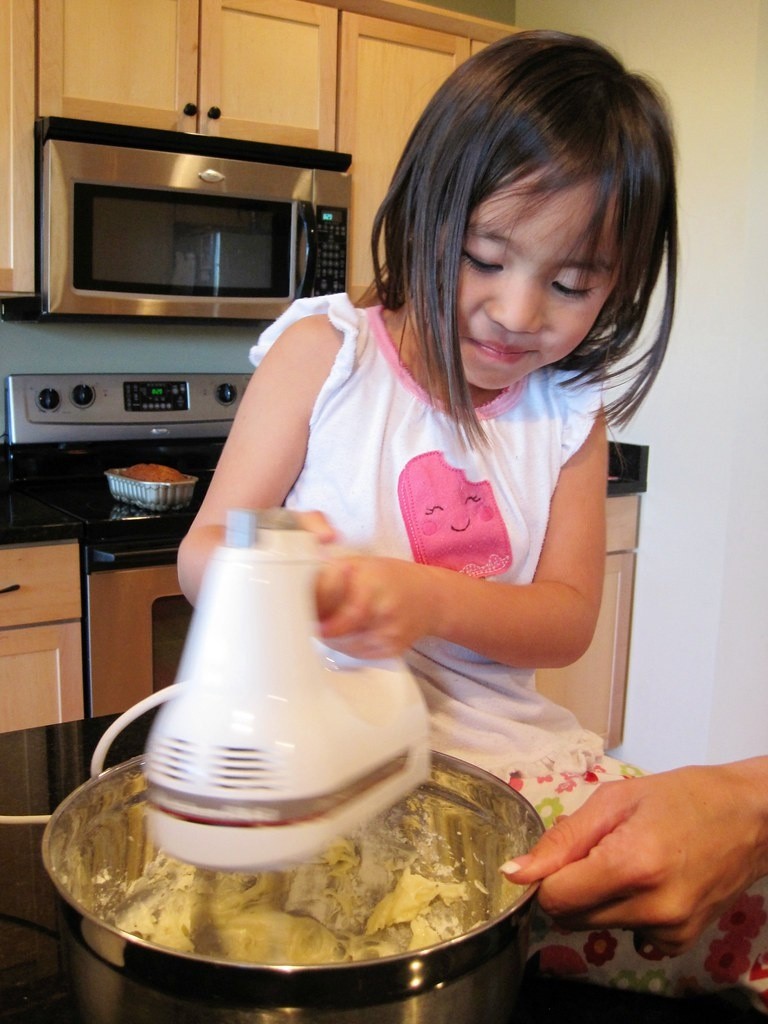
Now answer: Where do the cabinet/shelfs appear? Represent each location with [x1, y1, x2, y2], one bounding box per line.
[0, 0, 37, 299]
[35, 0, 340, 151]
[337, 0, 522, 306]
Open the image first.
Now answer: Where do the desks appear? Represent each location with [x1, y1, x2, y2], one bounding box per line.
[0, 711, 768, 1024]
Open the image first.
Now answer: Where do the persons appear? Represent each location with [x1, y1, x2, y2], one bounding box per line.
[175, 28, 768, 1017]
[495, 754, 768, 956]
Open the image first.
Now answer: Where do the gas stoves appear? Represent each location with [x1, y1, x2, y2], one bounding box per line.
[6, 373, 252, 545]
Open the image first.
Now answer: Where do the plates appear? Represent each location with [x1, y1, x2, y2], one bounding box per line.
[41, 750, 547, 1005]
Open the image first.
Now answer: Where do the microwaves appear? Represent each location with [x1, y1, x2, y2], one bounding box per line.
[34, 116, 352, 320]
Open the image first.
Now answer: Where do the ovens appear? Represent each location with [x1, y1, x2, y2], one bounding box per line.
[83, 539, 194, 718]
